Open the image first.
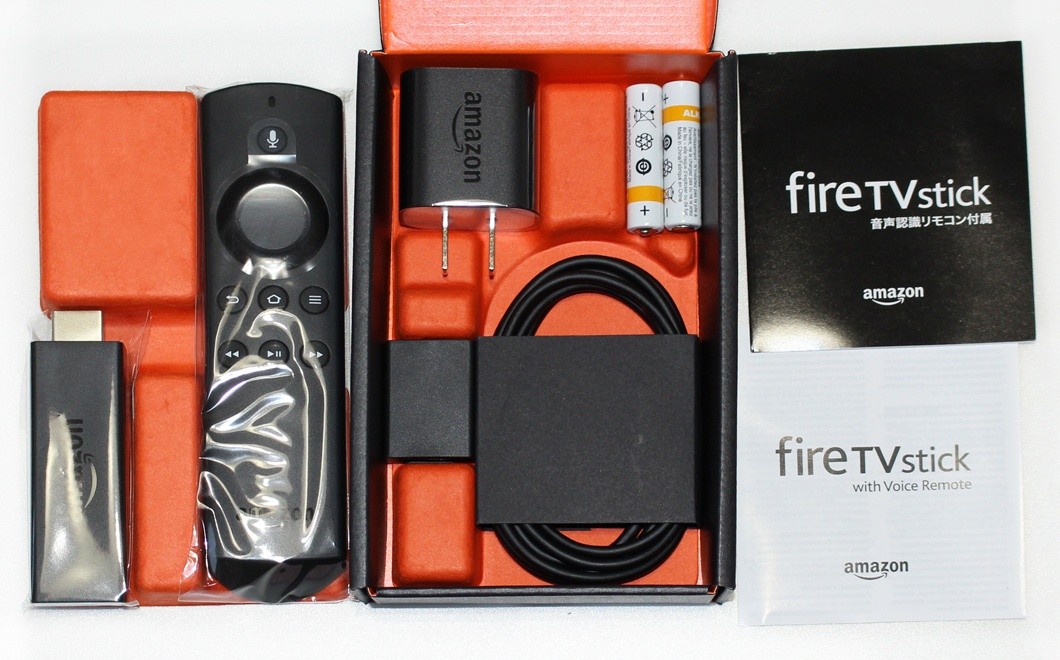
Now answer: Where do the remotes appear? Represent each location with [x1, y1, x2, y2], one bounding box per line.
[199, 80, 348, 600]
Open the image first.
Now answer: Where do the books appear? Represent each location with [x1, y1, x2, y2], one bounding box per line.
[733, 42, 1041, 621]
